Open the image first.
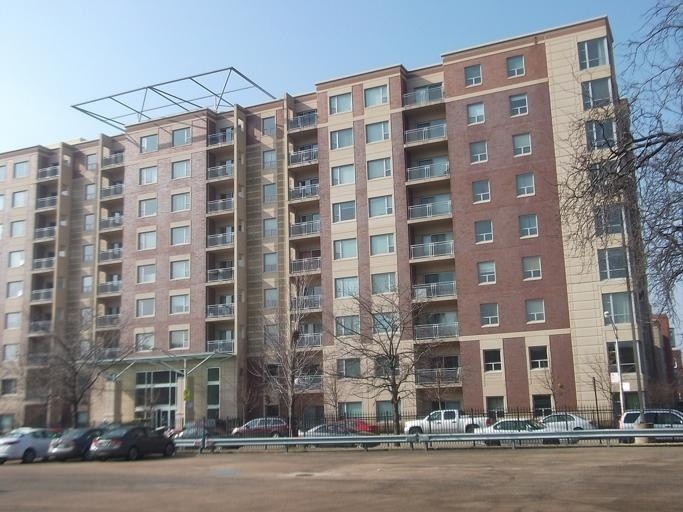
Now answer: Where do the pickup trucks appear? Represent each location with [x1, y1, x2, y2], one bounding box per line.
[404, 409, 493, 434]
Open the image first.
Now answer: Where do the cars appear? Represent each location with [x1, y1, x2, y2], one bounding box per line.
[474, 409, 682, 445]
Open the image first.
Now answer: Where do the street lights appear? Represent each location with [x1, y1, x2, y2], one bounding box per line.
[603, 311, 626, 415]
